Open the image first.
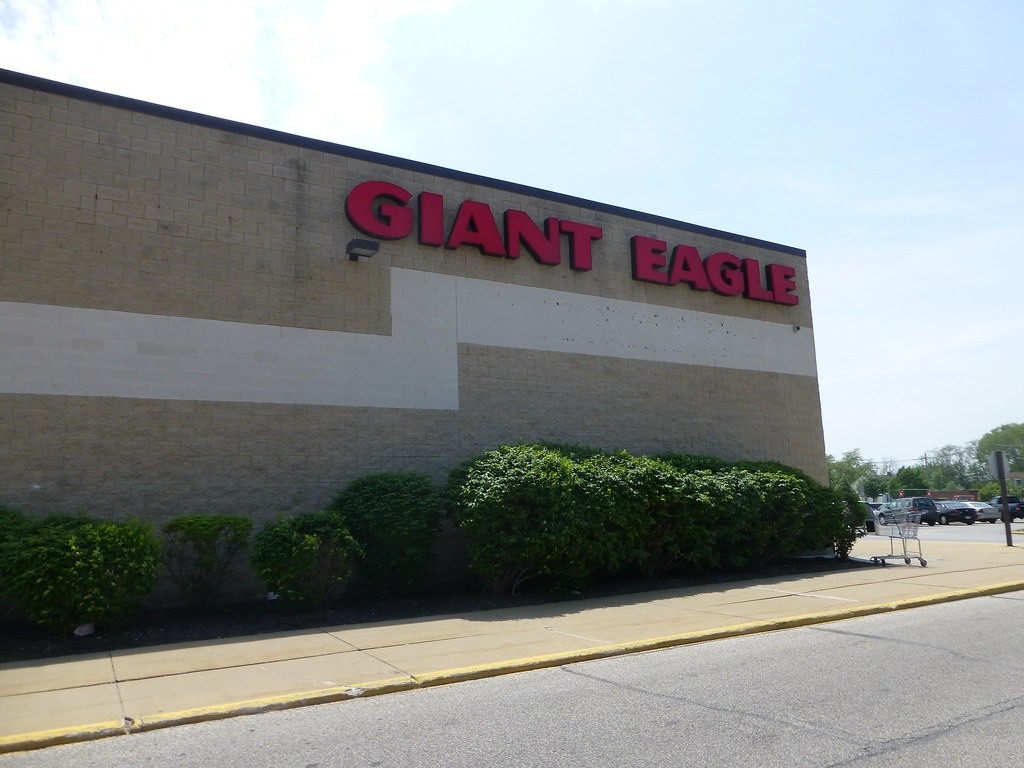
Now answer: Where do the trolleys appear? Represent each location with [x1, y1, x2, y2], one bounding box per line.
[870, 504, 928, 568]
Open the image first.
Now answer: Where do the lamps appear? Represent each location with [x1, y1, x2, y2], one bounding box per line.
[345, 238, 379, 261]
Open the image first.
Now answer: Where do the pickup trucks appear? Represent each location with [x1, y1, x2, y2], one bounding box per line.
[986, 495, 1024, 523]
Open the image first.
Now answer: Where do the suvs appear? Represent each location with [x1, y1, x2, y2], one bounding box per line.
[840, 500, 882, 533]
[878, 496, 937, 526]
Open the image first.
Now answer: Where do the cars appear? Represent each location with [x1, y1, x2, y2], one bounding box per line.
[960, 501, 1003, 524]
[934, 500, 976, 526]
